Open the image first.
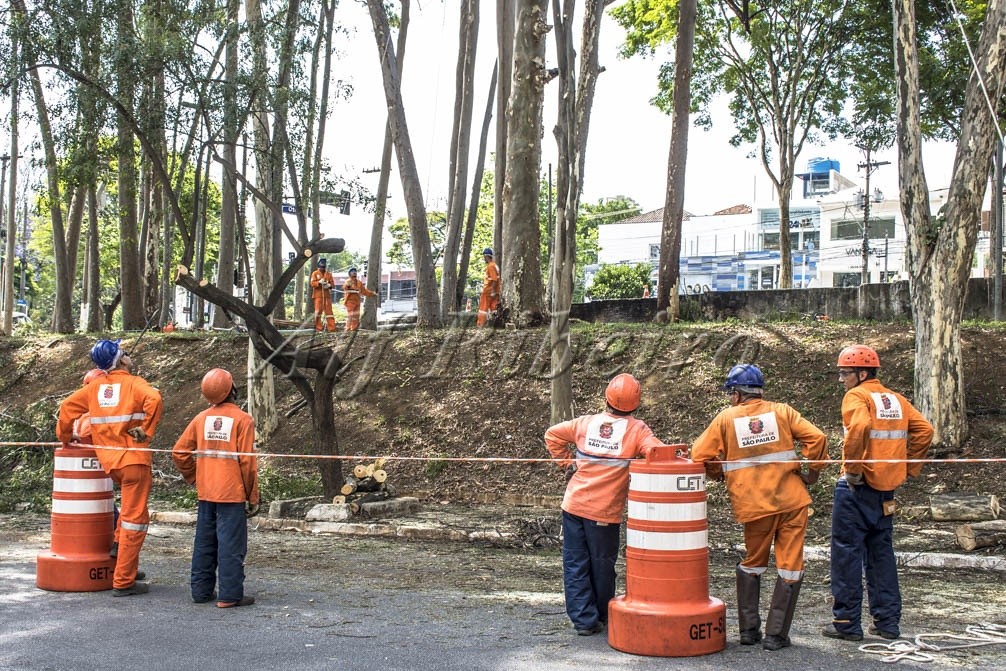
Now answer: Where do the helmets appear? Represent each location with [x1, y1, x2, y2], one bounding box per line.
[348, 269, 357, 276]
[201, 368, 233, 403]
[317, 259, 326, 266]
[605, 373, 641, 411]
[725, 364, 764, 386]
[91, 338, 120, 369]
[837, 345, 882, 367]
[483, 248, 493, 255]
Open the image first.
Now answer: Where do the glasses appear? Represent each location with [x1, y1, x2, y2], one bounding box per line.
[837, 371, 859, 376]
[122, 350, 130, 357]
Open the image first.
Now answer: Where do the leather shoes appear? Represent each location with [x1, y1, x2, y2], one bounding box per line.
[822, 623, 864, 641]
[868, 622, 898, 637]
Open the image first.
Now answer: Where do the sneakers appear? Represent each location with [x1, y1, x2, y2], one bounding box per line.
[216, 594, 255, 607]
[207, 591, 217, 600]
[578, 621, 603, 635]
[110, 543, 117, 557]
[112, 583, 147, 597]
[136, 572, 145, 580]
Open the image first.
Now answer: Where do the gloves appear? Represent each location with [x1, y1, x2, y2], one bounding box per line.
[327, 283, 331, 287]
[319, 278, 327, 285]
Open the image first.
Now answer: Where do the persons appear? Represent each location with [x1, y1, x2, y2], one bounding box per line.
[343, 268, 379, 331]
[821, 344, 935, 641]
[73, 368, 146, 579]
[544, 374, 669, 635]
[688, 361, 827, 650]
[59, 339, 163, 596]
[476, 248, 501, 328]
[310, 259, 336, 332]
[172, 368, 259, 608]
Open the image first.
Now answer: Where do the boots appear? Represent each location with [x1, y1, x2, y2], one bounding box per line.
[762, 575, 804, 650]
[736, 563, 763, 644]
